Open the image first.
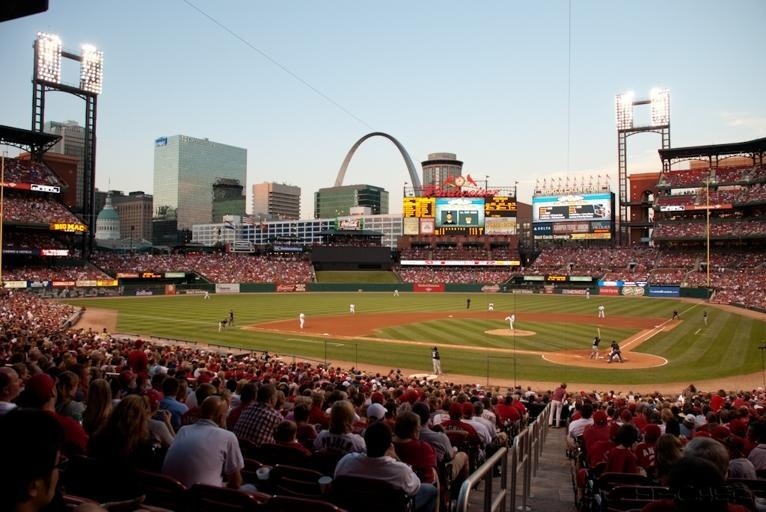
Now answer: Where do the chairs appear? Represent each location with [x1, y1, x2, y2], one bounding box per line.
[577, 452, 765, 511]
[60, 423, 517, 511]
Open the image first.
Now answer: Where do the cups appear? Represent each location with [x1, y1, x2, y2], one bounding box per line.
[256, 466, 270, 480]
[318, 476, 334, 491]
[755, 497, 766, 512]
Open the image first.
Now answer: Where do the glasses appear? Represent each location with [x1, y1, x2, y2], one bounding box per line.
[27, 453, 69, 476]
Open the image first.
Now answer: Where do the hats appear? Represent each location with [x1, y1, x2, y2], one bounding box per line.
[694, 419, 748, 449]
[399, 389, 417, 401]
[367, 402, 388, 420]
[134, 339, 146, 346]
[640, 423, 660, 438]
[684, 413, 696, 424]
[365, 422, 391, 458]
[448, 402, 474, 419]
[140, 389, 164, 406]
[592, 410, 608, 423]
[371, 392, 383, 402]
[620, 410, 633, 418]
[24, 374, 60, 401]
